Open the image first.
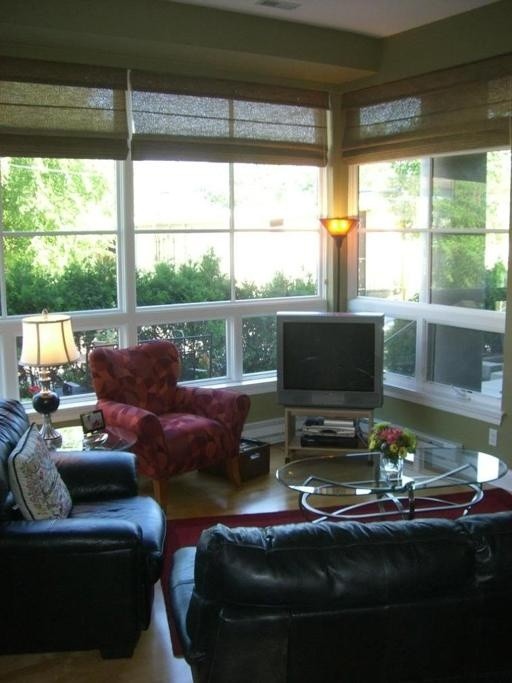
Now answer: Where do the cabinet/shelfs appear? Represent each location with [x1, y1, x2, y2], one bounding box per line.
[281, 407, 375, 466]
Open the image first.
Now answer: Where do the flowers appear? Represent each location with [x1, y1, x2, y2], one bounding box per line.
[364, 422, 421, 466]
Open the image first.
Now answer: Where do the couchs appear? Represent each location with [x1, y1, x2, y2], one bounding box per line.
[170, 512, 510, 683]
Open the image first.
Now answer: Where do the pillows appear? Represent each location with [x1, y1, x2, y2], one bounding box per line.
[9, 420, 74, 523]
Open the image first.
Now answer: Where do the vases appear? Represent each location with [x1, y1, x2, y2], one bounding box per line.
[378, 453, 403, 483]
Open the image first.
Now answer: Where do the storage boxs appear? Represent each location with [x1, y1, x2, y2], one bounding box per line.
[199, 438, 272, 481]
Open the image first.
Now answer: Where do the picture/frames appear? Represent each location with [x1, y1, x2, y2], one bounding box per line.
[81, 409, 106, 431]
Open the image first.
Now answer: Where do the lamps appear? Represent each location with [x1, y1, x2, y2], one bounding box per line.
[319, 216, 360, 312]
[18, 306, 83, 446]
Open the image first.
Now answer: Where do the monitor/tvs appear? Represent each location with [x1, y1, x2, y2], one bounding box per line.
[276, 311, 385, 408]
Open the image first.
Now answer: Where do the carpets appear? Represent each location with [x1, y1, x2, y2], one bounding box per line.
[161, 486, 511, 657]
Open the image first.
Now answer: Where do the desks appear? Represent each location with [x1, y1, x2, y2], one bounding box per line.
[48, 421, 138, 452]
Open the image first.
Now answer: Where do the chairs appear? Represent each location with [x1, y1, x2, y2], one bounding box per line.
[1, 397, 168, 662]
[89, 340, 251, 513]
